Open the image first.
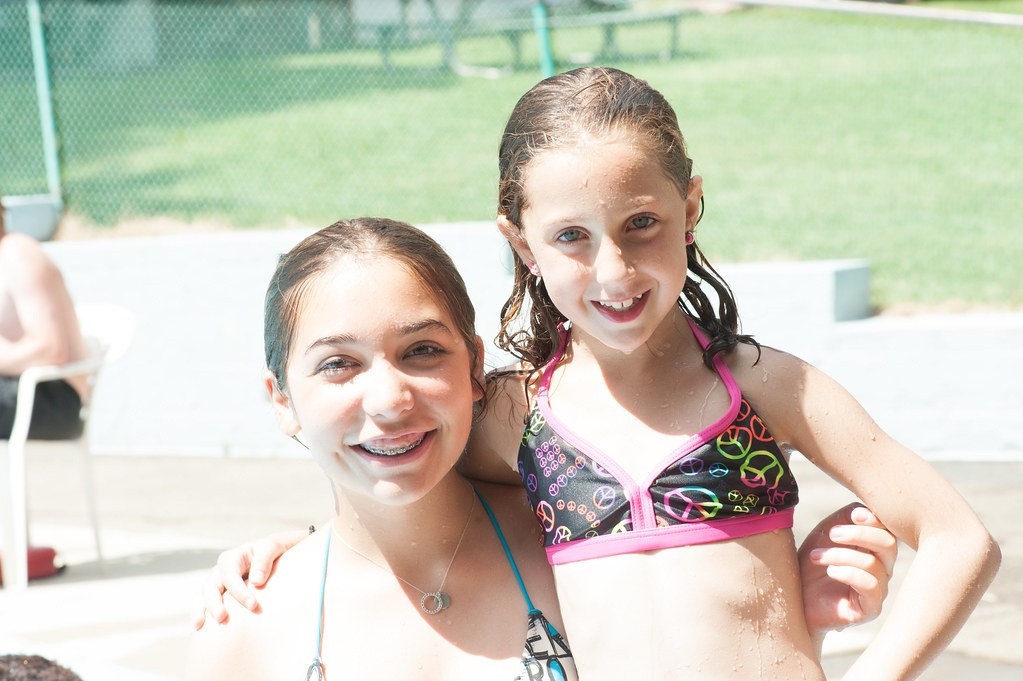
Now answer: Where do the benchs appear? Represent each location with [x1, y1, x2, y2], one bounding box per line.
[363, 10, 699, 72]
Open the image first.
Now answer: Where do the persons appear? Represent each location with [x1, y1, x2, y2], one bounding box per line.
[180, 215, 898, 681]
[193, 68, 1002, 681]
[0, 205, 85, 441]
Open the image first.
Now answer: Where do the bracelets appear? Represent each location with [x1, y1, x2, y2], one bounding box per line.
[309, 525, 316, 535]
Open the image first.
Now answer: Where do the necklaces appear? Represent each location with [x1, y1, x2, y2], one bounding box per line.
[328, 488, 477, 614]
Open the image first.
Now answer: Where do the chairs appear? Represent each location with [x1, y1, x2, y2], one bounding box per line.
[0, 341, 107, 592]
